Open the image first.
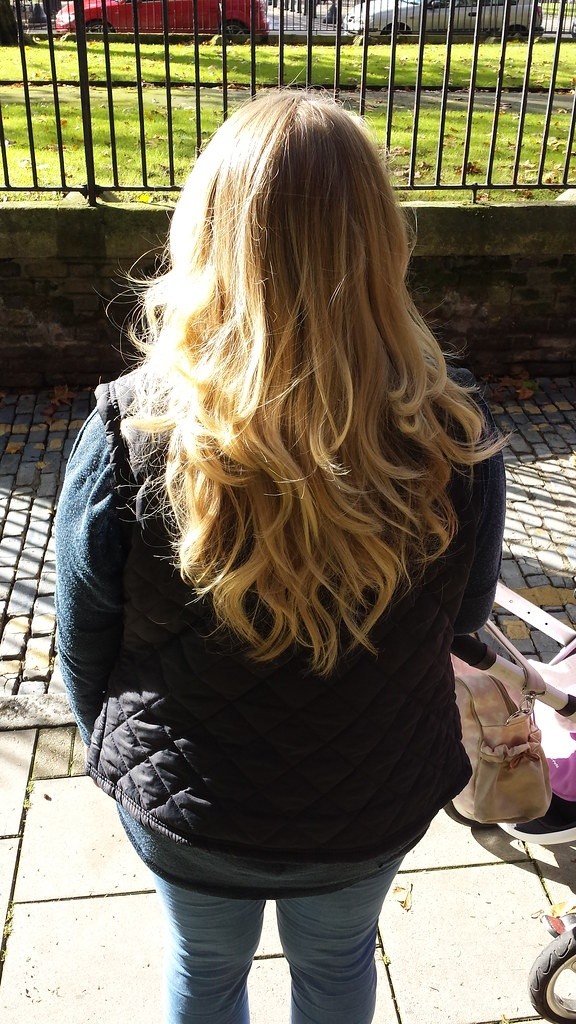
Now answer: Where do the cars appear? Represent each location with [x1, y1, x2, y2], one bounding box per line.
[55, 0, 269, 34]
[343, 0, 544, 35]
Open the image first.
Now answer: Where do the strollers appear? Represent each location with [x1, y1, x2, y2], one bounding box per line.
[441, 580, 576, 1024]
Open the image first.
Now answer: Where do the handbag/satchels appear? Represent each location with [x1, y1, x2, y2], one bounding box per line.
[449, 634, 554, 825]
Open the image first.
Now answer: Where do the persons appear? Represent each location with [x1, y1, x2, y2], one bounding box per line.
[53, 92, 507, 1024]
